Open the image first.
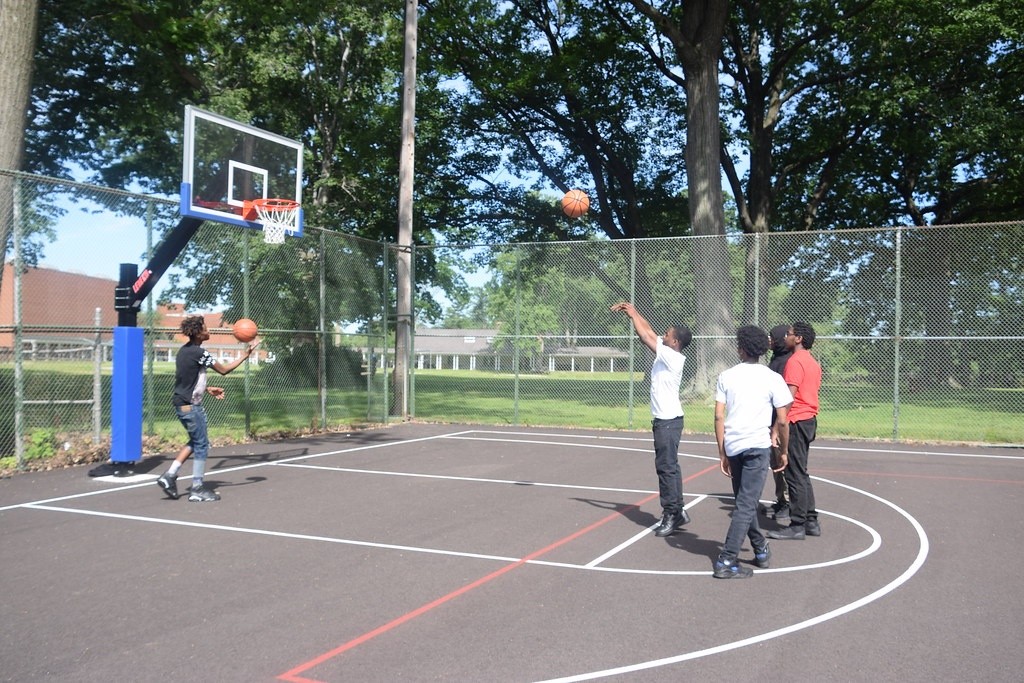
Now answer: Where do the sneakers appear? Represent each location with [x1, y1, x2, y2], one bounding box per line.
[773, 504, 790, 520]
[761, 504, 777, 514]
[755, 543, 771, 568]
[805, 524, 821, 536]
[766, 524, 806, 540]
[655, 512, 685, 536]
[712, 558, 753, 579]
[676, 510, 690, 527]
[188, 484, 220, 501]
[156, 473, 178, 500]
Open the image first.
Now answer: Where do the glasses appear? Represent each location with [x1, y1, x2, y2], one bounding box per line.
[785, 333, 796, 338]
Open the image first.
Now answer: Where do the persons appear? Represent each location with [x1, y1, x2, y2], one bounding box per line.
[611, 300, 693, 538]
[712, 325, 794, 580]
[155, 315, 263, 502]
[760, 320, 822, 540]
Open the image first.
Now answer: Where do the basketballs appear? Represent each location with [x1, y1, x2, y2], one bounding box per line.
[232, 318, 258, 343]
[561, 190, 590, 218]
